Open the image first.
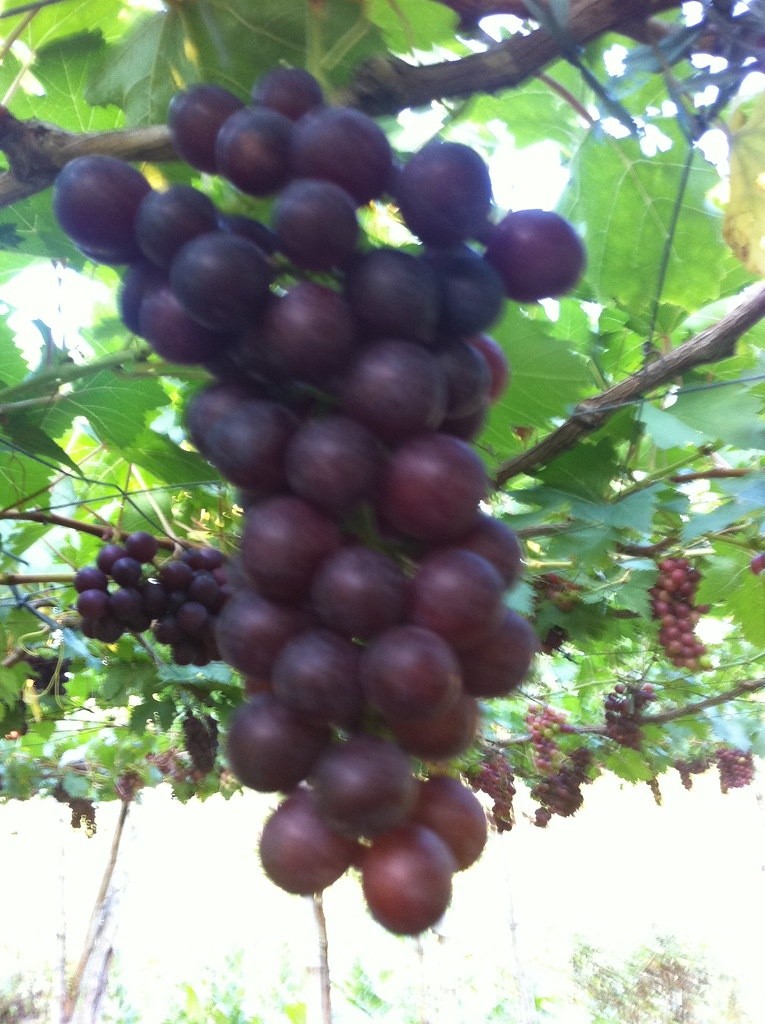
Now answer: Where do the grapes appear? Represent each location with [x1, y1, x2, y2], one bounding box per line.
[52, 66, 757, 938]
